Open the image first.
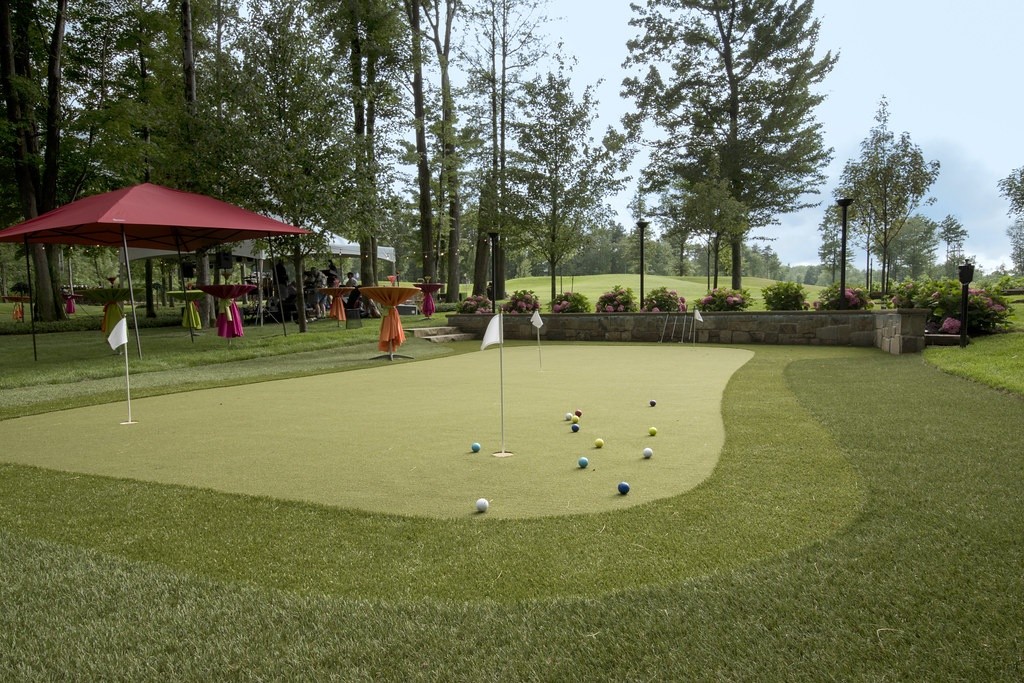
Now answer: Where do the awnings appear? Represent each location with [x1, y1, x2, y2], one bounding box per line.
[119, 198, 396, 309]
[1, 183, 315, 360]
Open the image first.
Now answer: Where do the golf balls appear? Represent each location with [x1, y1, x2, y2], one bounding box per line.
[572, 416, 579, 422]
[649, 427, 657, 435]
[595, 438, 604, 447]
[643, 448, 652, 457]
[575, 409, 582, 416]
[566, 413, 572, 420]
[572, 424, 579, 431]
[618, 482, 629, 494]
[579, 457, 588, 467]
[476, 498, 488, 510]
[650, 400, 656, 406]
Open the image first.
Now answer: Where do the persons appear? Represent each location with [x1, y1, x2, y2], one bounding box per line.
[246, 260, 359, 319]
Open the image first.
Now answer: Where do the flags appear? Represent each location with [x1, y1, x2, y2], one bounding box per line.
[694, 309, 703, 322]
[481, 314, 503, 351]
[530, 311, 543, 328]
[108, 317, 128, 350]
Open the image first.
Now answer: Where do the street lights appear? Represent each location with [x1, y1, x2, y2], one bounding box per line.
[636, 220, 653, 311]
[836, 198, 853, 309]
[958, 259, 975, 348]
[487, 231, 500, 312]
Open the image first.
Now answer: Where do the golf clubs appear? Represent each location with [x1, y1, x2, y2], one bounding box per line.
[679, 304, 688, 343]
[658, 313, 669, 343]
[687, 316, 693, 341]
[671, 314, 678, 340]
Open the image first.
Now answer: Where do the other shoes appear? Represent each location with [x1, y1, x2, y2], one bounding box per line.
[316, 313, 326, 319]
